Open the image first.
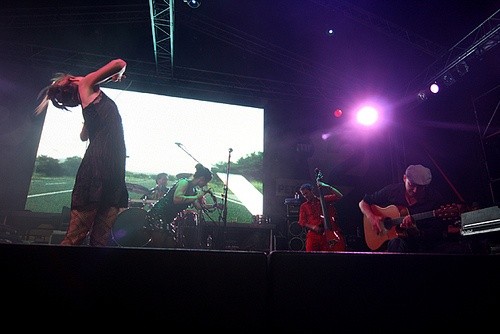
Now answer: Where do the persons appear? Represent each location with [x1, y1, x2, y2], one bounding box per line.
[148, 163, 225, 222]
[46, 59, 128, 245]
[358, 164, 448, 252]
[141, 173, 169, 200]
[297, 182, 347, 251]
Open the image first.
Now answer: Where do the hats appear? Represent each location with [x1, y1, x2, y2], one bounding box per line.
[405, 164, 432, 186]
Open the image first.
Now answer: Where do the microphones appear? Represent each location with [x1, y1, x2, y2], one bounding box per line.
[229, 148, 233, 152]
[175, 142, 182, 145]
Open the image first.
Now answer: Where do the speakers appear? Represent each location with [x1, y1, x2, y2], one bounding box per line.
[287, 202, 306, 251]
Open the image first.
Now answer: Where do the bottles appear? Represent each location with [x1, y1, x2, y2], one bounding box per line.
[206, 234, 213, 246]
[295, 192, 299, 199]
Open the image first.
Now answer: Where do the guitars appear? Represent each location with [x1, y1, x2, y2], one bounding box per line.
[363, 200, 460, 251]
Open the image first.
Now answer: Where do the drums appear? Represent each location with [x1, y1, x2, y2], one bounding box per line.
[175, 210, 198, 228]
[110, 207, 152, 248]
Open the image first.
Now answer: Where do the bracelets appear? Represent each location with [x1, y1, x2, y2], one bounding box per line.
[327, 186, 332, 192]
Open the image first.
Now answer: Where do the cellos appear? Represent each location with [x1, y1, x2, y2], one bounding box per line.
[314, 167, 346, 252]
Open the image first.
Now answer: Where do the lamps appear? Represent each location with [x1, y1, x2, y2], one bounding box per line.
[416, 55, 477, 104]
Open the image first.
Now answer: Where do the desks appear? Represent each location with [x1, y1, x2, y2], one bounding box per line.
[206, 222, 277, 253]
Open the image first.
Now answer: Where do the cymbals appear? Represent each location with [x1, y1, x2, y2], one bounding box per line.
[125, 182, 150, 195]
[175, 172, 194, 179]
